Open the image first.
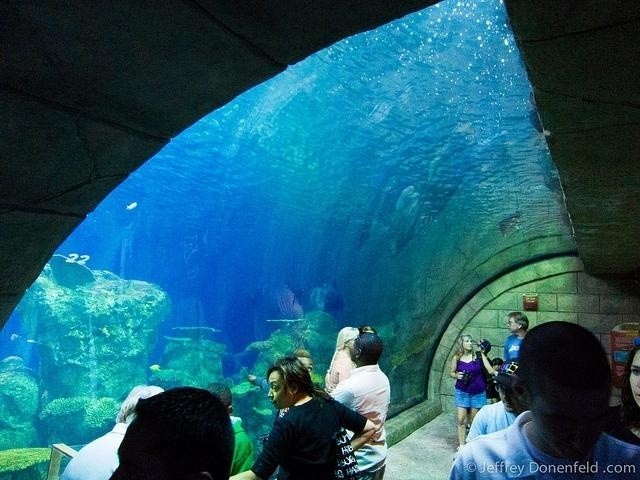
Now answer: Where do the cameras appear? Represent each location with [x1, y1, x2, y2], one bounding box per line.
[461, 370, 471, 386]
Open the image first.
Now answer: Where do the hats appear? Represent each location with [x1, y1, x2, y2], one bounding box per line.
[489, 358, 520, 385]
[474, 338, 492, 355]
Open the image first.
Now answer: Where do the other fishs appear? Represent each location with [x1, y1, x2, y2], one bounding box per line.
[498, 211, 522, 237]
[0, 355, 24, 371]
[149, 364, 162, 375]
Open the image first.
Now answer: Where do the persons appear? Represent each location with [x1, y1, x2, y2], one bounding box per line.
[56, 383, 165, 480]
[246, 349, 314, 480]
[229, 354, 374, 480]
[360, 325, 377, 335]
[449, 310, 639, 479]
[322, 325, 360, 394]
[327, 332, 392, 479]
[202, 381, 255, 480]
[106, 384, 235, 480]
